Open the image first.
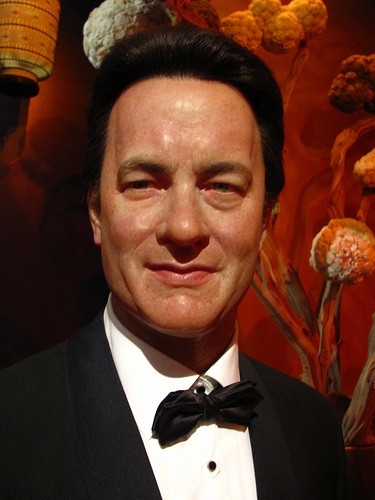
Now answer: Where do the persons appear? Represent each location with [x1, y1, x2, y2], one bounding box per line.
[0, 22, 348, 500]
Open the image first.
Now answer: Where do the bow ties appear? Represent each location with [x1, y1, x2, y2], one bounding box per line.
[151, 379, 264, 447]
